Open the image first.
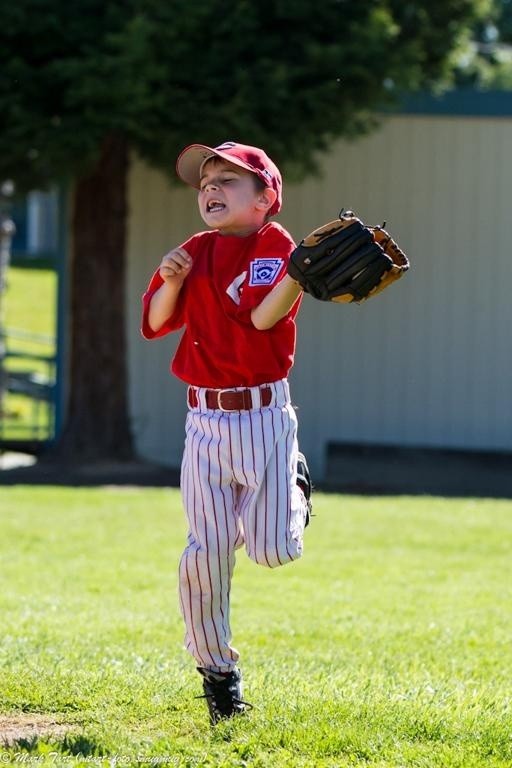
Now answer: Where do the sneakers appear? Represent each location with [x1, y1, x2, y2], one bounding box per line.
[296, 451, 312, 529]
[195, 667, 247, 729]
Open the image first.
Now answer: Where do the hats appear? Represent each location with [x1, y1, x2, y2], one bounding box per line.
[175, 141, 282, 216]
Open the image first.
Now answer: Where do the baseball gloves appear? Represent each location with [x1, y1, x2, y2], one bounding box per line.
[286, 209, 410, 305]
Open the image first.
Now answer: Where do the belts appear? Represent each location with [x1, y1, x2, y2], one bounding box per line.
[188, 387, 272, 411]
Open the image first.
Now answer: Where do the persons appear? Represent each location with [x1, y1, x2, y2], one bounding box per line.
[140, 142, 311, 729]
